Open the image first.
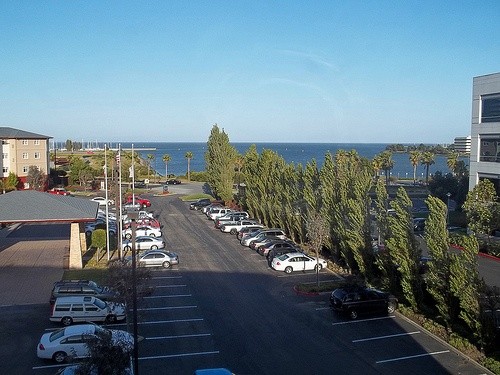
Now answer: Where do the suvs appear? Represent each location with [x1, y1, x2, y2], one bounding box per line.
[269, 248, 304, 263]
[214, 211, 250, 223]
[238, 227, 264, 240]
[329, 287, 399, 319]
[119, 202, 141, 210]
[49, 279, 121, 304]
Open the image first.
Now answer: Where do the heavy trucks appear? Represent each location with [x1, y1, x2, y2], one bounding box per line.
[17, 177, 70, 196]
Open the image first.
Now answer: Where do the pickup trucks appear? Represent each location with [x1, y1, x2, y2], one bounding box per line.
[190, 199, 225, 211]
[215, 215, 257, 228]
[206, 206, 236, 216]
[220, 220, 264, 235]
[117, 196, 151, 208]
[210, 207, 239, 220]
[123, 211, 154, 220]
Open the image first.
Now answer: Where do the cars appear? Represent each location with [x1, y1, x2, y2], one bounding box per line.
[123, 216, 161, 229]
[89, 197, 115, 206]
[271, 252, 328, 274]
[249, 236, 292, 251]
[123, 236, 165, 251]
[259, 242, 301, 257]
[413, 217, 427, 231]
[126, 250, 179, 269]
[84, 209, 117, 236]
[193, 368, 240, 375]
[202, 203, 230, 214]
[122, 225, 162, 239]
[166, 179, 181, 185]
[37, 324, 134, 363]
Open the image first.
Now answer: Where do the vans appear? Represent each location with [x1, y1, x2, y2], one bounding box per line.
[241, 228, 287, 247]
[49, 296, 127, 325]
